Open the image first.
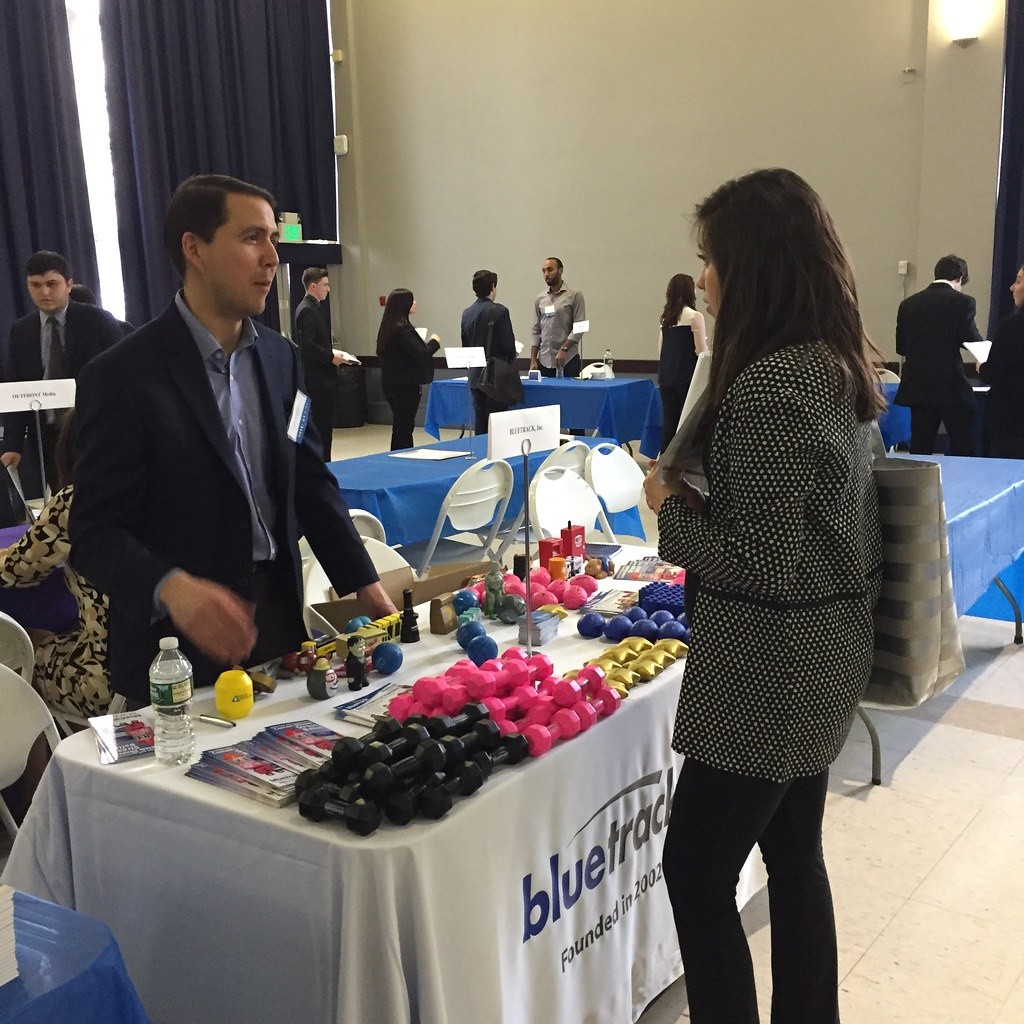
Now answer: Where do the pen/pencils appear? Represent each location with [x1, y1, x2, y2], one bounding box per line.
[192, 712, 237, 732]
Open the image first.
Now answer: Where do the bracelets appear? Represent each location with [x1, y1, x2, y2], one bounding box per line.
[560, 346, 568, 352]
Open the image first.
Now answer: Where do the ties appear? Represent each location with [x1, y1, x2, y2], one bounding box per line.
[50, 318, 66, 433]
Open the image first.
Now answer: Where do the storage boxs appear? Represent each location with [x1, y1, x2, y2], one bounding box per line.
[307, 561, 493, 640]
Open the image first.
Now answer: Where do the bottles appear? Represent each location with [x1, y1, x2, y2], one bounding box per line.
[639, 581, 684, 620]
[604, 349, 613, 370]
[148, 637, 195, 765]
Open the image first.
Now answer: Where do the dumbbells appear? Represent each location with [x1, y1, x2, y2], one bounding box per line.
[294, 646, 621, 837]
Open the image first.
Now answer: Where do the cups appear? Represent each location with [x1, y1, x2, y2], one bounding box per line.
[556, 366, 563, 378]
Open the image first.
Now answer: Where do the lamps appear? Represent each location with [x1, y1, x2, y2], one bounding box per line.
[279, 211, 300, 224]
[953, 37, 978, 50]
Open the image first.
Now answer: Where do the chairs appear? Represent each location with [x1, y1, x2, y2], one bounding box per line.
[296, 440, 650, 640]
[579, 362, 616, 438]
[0, 612, 126, 844]
[875, 368, 901, 384]
[6, 464, 53, 525]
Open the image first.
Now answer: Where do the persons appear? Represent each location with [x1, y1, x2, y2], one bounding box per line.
[982, 264, 1024, 459]
[894, 254, 984, 457]
[376, 288, 440, 451]
[69, 176, 397, 714]
[658, 274, 708, 456]
[346, 635, 369, 690]
[0, 406, 107, 777]
[295, 268, 346, 462]
[485, 562, 502, 620]
[530, 257, 585, 378]
[643, 169, 889, 1024]
[0, 250, 136, 500]
[461, 270, 516, 437]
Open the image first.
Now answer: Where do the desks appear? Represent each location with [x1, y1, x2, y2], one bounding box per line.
[332, 363, 365, 428]
[0, 543, 769, 1024]
[857, 455, 1024, 785]
[0, 884, 151, 1024]
[424, 378, 654, 458]
[325, 433, 646, 550]
[0, 525, 80, 643]
[639, 383, 912, 459]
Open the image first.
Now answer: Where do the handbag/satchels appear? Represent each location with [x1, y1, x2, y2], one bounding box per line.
[480, 355, 526, 408]
[863, 457, 966, 709]
